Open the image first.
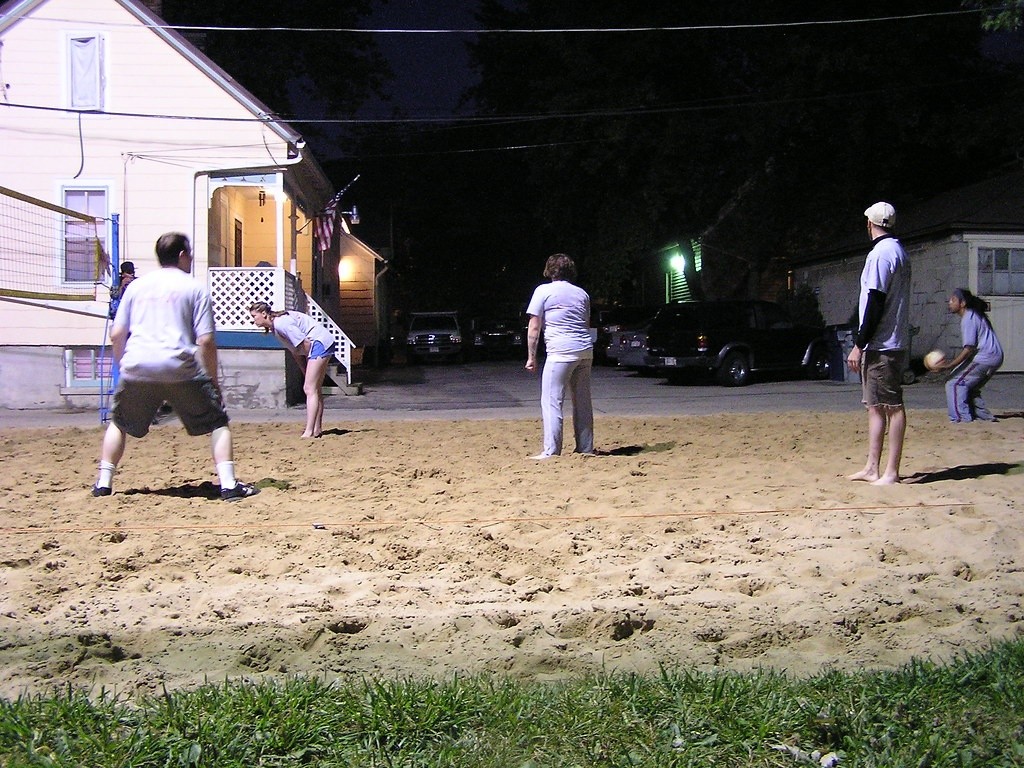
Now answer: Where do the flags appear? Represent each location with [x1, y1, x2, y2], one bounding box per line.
[314, 181, 354, 251]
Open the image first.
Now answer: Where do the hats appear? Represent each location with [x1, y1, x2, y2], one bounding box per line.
[121, 262, 138, 273]
[864, 202, 895, 229]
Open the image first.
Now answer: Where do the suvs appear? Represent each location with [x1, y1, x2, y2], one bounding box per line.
[406, 313, 463, 360]
[472, 316, 522, 354]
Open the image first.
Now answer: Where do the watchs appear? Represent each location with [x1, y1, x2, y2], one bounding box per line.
[942, 365, 946, 371]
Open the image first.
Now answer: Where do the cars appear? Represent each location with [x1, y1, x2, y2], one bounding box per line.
[603, 298, 834, 388]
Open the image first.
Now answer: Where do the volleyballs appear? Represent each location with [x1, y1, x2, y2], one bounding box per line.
[924, 348, 947, 373]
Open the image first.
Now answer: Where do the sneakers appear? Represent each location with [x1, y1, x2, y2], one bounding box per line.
[91, 484, 111, 496]
[221, 480, 260, 504]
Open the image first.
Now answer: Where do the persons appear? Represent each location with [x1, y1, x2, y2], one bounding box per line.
[249, 301, 337, 440]
[845, 202, 912, 487]
[92, 231, 258, 503]
[930, 287, 1003, 424]
[525, 253, 595, 461]
